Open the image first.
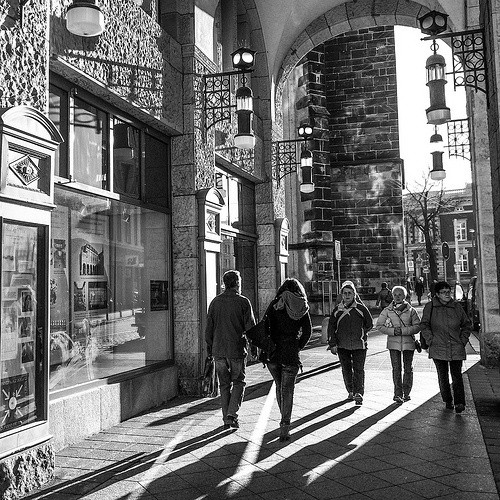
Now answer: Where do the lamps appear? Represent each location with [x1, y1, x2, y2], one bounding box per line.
[430, 118, 471, 180]
[203, 47, 256, 148]
[65, 0, 105, 37]
[272, 125, 315, 193]
[418, 10, 489, 126]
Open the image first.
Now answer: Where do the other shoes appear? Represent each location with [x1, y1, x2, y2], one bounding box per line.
[280, 424, 291, 442]
[404, 396, 411, 400]
[280, 421, 285, 428]
[224, 424, 230, 428]
[354, 393, 363, 405]
[225, 414, 239, 428]
[348, 394, 355, 400]
[393, 396, 404, 403]
[455, 404, 465, 413]
[445, 402, 454, 409]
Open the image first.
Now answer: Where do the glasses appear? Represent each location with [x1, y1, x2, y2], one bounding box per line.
[440, 290, 451, 295]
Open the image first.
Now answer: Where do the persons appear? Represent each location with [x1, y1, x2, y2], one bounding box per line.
[415, 279, 425, 306]
[258, 277, 313, 442]
[421, 281, 472, 413]
[375, 282, 393, 311]
[328, 281, 373, 406]
[376, 286, 422, 403]
[204, 270, 258, 429]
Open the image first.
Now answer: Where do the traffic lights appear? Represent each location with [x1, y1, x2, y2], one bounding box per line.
[442, 242, 450, 260]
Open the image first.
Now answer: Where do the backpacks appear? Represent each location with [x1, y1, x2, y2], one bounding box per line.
[385, 289, 393, 303]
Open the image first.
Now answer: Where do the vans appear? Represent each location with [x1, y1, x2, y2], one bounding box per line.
[454, 276, 480, 331]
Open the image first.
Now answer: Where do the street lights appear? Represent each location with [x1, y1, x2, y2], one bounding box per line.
[469, 229, 476, 276]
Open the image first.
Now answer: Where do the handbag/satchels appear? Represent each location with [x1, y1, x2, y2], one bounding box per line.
[415, 340, 421, 353]
[420, 332, 429, 349]
[246, 317, 270, 350]
[201, 355, 218, 397]
